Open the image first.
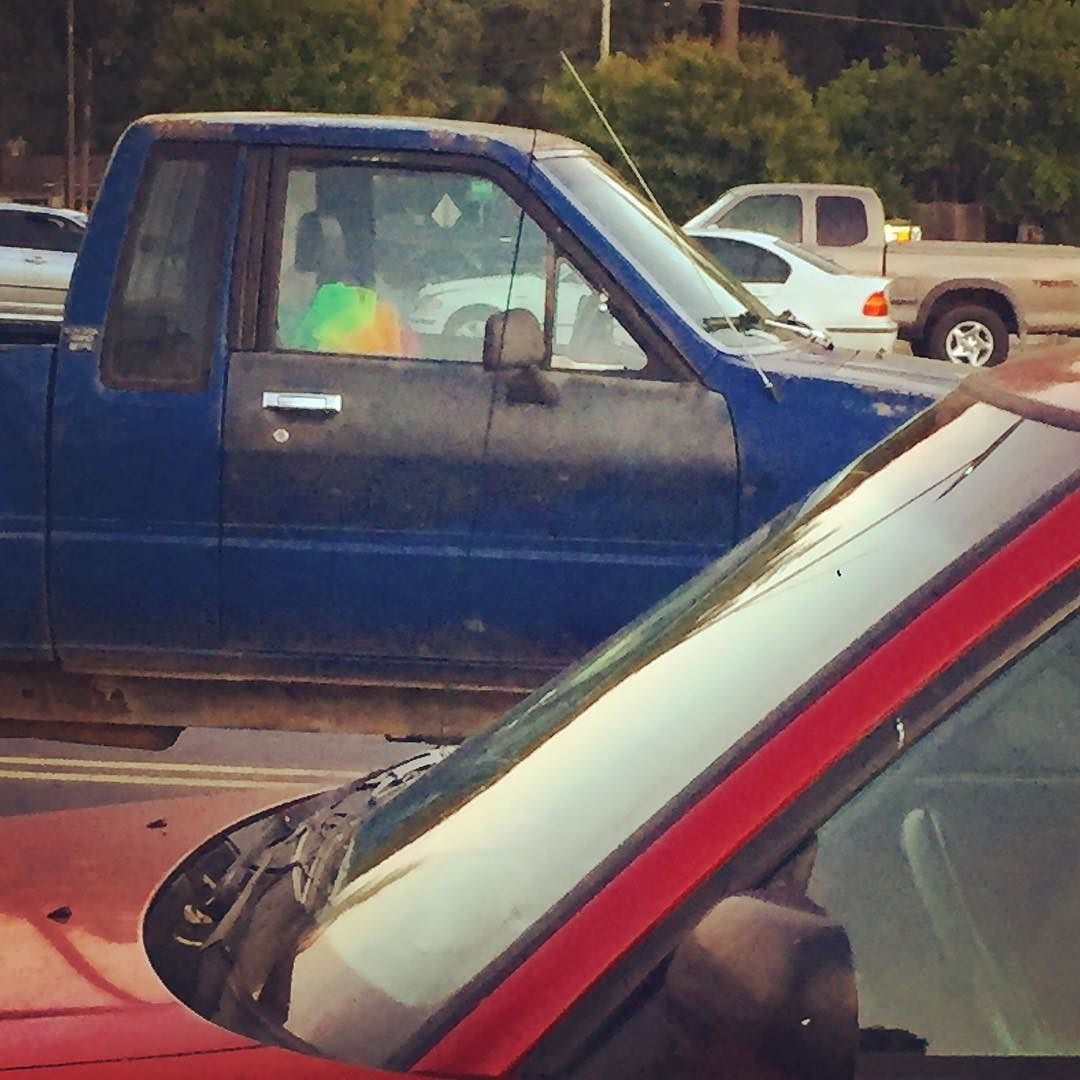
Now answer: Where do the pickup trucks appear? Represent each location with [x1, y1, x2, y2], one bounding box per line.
[679, 183, 1080, 372]
[0, 110, 994, 751]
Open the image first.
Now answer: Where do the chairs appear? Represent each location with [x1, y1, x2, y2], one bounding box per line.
[284, 210, 360, 336]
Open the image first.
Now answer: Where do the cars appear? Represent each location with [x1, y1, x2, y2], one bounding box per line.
[407, 222, 901, 353]
[0, 332, 1080, 1079]
[1, 201, 90, 321]
